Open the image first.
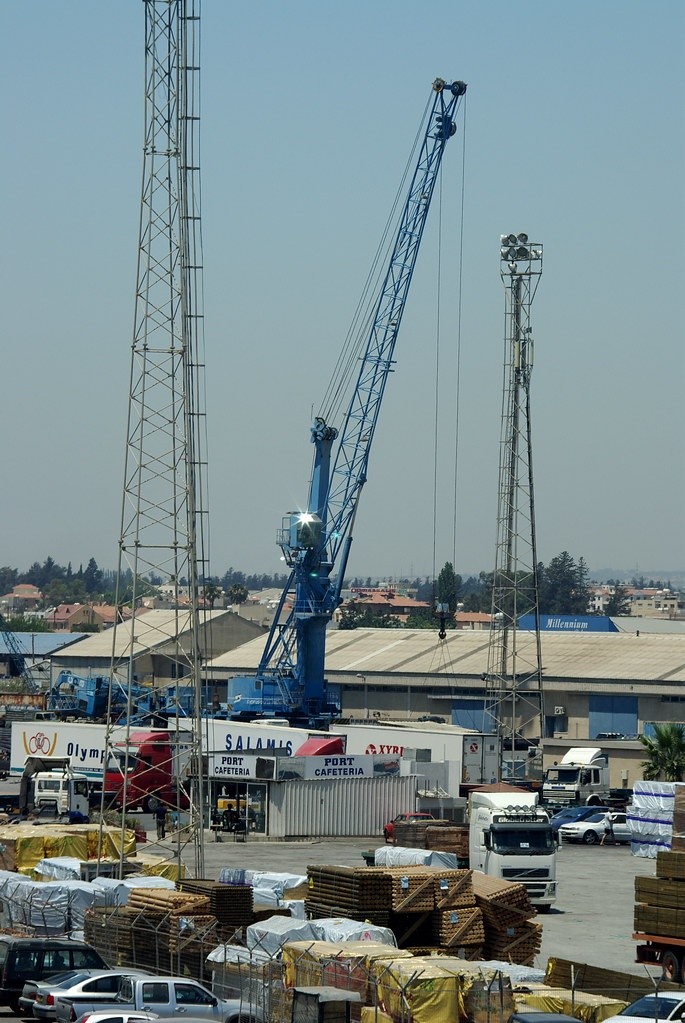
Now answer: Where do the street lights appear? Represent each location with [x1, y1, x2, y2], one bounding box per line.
[356, 673, 368, 720]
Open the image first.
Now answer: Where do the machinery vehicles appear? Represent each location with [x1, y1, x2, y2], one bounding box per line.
[44, 670, 224, 726]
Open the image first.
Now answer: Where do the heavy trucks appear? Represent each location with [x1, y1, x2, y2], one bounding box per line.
[101, 726, 348, 814]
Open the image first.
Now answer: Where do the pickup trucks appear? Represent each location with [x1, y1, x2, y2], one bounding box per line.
[55, 976, 267, 1023]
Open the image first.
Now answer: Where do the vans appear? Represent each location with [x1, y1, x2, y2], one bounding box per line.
[0, 934, 114, 1016]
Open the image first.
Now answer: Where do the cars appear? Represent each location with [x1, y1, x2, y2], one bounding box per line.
[552, 803, 622, 841]
[72, 1009, 162, 1023]
[600, 991, 685, 1023]
[382, 812, 437, 842]
[32, 968, 183, 1018]
[506, 1011, 584, 1023]
[558, 810, 633, 846]
[17, 963, 159, 1010]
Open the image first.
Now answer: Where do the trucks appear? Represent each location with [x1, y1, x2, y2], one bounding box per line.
[0, 753, 90, 826]
[540, 746, 633, 814]
[630, 930, 685, 986]
[361, 790, 561, 915]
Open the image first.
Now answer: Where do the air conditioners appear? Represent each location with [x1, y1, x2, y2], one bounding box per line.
[554, 705, 566, 715]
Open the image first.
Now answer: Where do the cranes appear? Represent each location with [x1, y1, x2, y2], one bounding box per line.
[223, 77, 470, 734]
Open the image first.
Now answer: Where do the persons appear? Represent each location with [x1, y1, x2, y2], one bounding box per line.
[600, 807, 620, 846]
[152, 802, 167, 840]
[221, 803, 255, 830]
[165, 809, 174, 833]
